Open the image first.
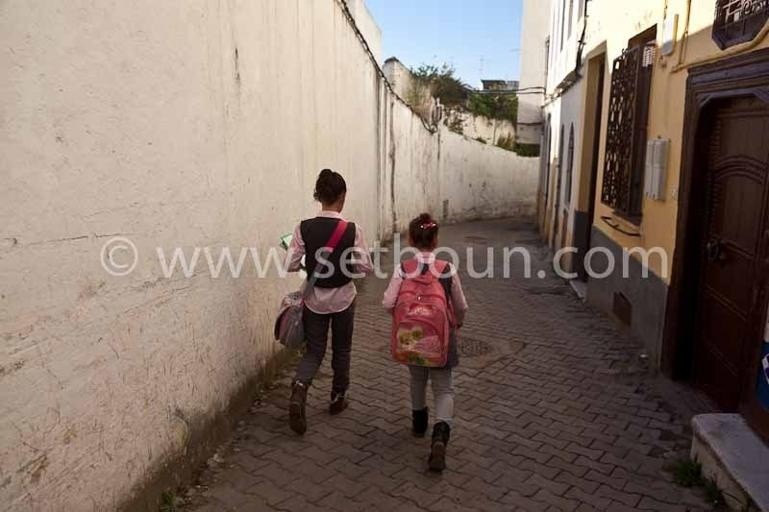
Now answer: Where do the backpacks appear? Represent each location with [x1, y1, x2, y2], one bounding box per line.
[390, 259, 451, 369]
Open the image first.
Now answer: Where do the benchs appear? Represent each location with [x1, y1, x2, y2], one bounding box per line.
[690, 411, 769, 512]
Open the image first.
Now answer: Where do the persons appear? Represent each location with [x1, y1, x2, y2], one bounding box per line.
[382, 213, 469, 471]
[282, 168, 372, 436]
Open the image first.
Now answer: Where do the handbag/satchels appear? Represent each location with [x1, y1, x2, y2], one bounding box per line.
[273, 291, 307, 350]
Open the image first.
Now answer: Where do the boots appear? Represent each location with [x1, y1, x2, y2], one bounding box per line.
[288, 383, 307, 434]
[329, 391, 349, 415]
[428, 421, 450, 471]
[411, 407, 429, 437]
